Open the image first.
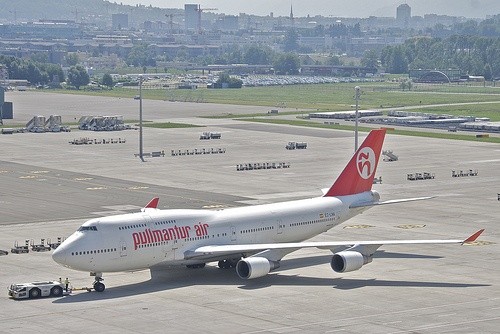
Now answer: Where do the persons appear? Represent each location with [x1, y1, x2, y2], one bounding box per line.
[58, 277, 62, 283]
[65, 277, 70, 292]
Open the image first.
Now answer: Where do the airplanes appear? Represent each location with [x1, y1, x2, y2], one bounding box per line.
[53, 128, 484, 292]
